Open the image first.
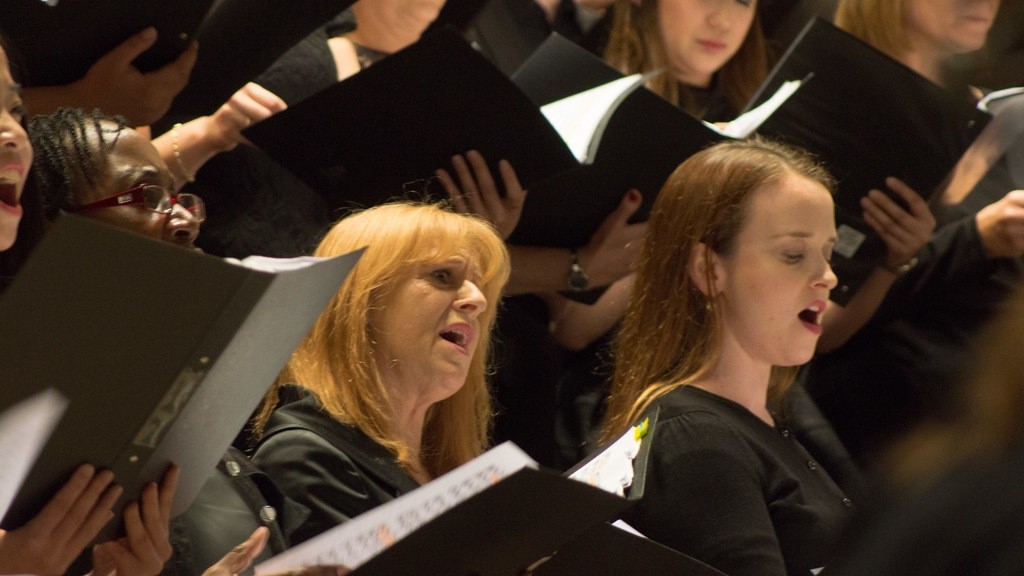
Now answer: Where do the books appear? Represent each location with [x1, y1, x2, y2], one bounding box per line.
[255, 406, 658, 576]
[0, 215, 369, 546]
[242, 37, 665, 211]
[530, 519, 722, 575]
[506, 34, 732, 246]
[1, 0, 215, 81]
[749, 15, 1024, 306]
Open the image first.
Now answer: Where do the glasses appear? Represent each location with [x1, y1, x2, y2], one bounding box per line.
[67, 181, 207, 225]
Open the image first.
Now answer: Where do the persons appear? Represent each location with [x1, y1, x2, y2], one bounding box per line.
[251, 203, 510, 547]
[605, 143, 866, 576]
[0, 0, 1024, 576]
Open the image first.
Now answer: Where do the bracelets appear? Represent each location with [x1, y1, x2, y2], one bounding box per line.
[173, 123, 195, 182]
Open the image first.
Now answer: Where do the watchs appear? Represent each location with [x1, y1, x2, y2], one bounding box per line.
[570, 246, 589, 290]
[881, 254, 919, 274]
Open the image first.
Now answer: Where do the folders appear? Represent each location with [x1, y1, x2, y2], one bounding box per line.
[0, 209, 368, 547]
[527, 521, 729, 575]
[240, 26, 645, 208]
[1, 0, 214, 72]
[744, 15, 1024, 310]
[510, 32, 815, 306]
[345, 404, 662, 576]
